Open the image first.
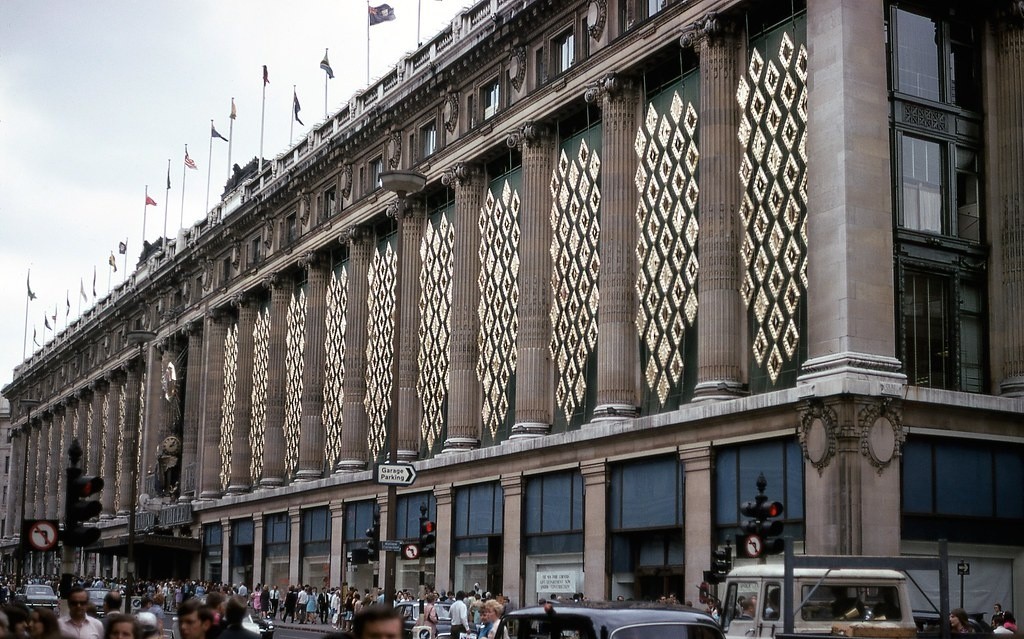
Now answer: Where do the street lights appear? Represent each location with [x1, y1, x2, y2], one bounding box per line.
[124, 329, 157, 614]
[9, 399, 43, 597]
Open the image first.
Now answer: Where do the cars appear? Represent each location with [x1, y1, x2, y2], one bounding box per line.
[392, 600, 478, 639]
[492, 600, 725, 639]
[912, 610, 995, 639]
[14, 583, 59, 616]
[83, 586, 112, 619]
[170, 597, 274, 639]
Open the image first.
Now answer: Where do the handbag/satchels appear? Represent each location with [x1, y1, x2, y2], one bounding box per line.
[427, 608, 438, 622]
[459, 631, 477, 639]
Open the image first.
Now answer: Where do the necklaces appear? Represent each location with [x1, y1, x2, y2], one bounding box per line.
[70, 619, 84, 638]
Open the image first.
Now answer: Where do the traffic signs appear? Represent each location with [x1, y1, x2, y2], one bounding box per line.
[372, 463, 417, 486]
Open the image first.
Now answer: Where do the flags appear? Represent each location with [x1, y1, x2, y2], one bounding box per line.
[320, 52, 335, 79]
[80, 267, 97, 303]
[185, 149, 198, 170]
[262, 65, 270, 86]
[293, 91, 304, 126]
[369, 3, 396, 26]
[27, 271, 37, 301]
[211, 124, 228, 142]
[52, 294, 70, 323]
[109, 241, 126, 272]
[45, 314, 52, 330]
[229, 102, 237, 120]
[34, 327, 40, 346]
[146, 167, 171, 206]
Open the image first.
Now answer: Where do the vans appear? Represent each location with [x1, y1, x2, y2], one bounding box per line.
[717, 564, 919, 639]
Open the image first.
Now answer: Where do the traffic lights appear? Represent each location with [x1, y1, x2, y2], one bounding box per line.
[756, 500, 786, 555]
[711, 550, 728, 582]
[419, 520, 436, 558]
[739, 501, 760, 536]
[62, 467, 105, 547]
[366, 524, 381, 560]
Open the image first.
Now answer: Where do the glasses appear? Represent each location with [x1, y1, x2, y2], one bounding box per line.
[69, 600, 86, 606]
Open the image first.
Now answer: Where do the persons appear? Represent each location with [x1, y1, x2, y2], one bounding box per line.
[177, 591, 262, 639]
[0, 585, 168, 639]
[250, 582, 780, 639]
[949, 604, 1018, 634]
[0, 569, 248, 612]
